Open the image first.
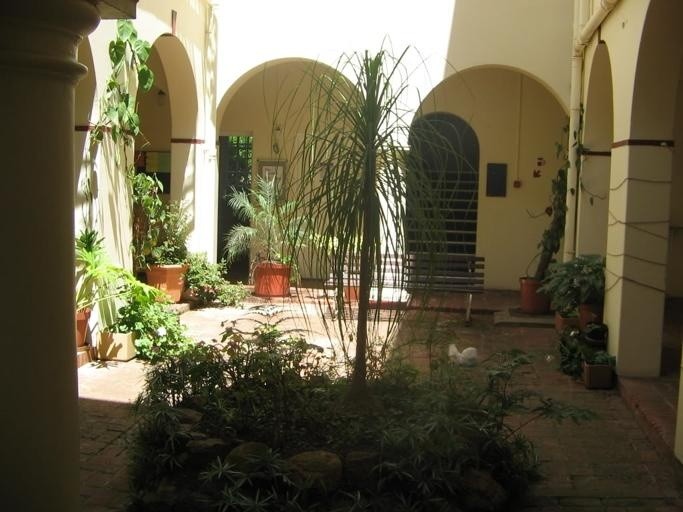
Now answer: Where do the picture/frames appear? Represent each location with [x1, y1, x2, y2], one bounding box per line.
[257, 160, 287, 201]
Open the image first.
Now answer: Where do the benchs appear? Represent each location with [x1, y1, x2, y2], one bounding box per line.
[323, 252, 486, 328]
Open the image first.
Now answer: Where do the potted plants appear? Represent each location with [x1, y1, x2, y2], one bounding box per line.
[75, 172, 192, 362]
[519, 167, 615, 390]
[223, 170, 308, 297]
